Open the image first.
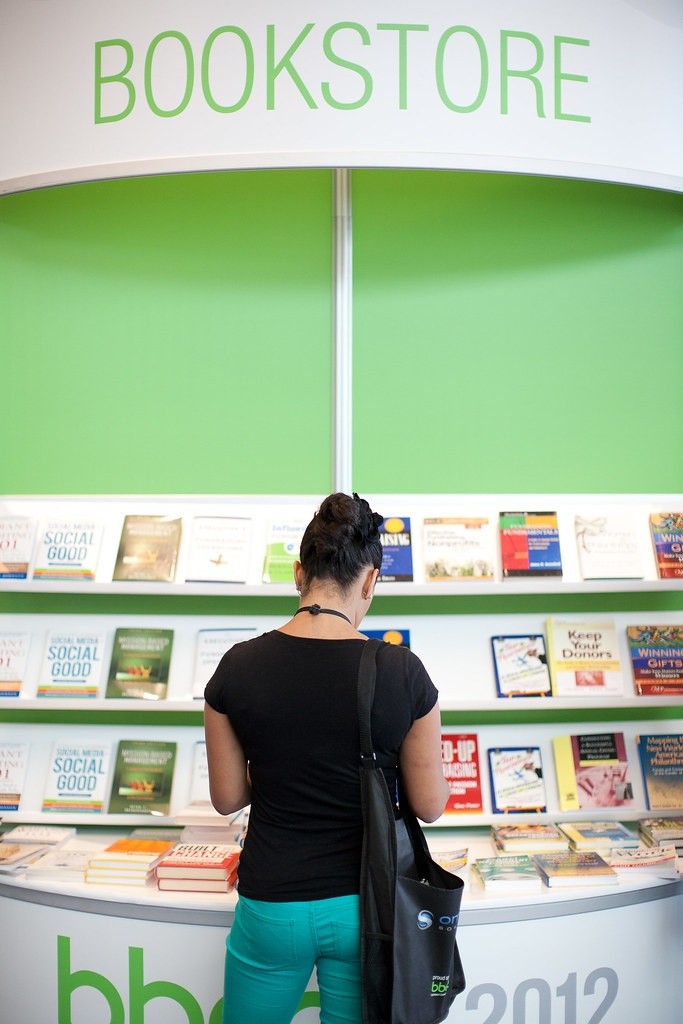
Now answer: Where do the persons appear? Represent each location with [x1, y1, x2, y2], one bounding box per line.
[202, 493, 450, 1024]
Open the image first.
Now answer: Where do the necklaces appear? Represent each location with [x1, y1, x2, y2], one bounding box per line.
[294, 605, 352, 625]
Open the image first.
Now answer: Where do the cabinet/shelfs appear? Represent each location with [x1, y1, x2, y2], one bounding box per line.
[0, 491, 683, 827]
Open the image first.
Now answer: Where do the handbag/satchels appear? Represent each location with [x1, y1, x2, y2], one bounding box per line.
[357, 638, 465, 1024]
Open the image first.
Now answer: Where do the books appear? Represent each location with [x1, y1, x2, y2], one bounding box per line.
[371, 517, 413, 582]
[429, 732, 683, 893]
[423, 517, 496, 581]
[491, 616, 683, 697]
[0, 737, 252, 825]
[649, 511, 683, 580]
[574, 513, 643, 579]
[0, 627, 257, 701]
[0, 508, 314, 585]
[0, 823, 247, 894]
[499, 511, 562, 581]
[359, 629, 410, 650]
